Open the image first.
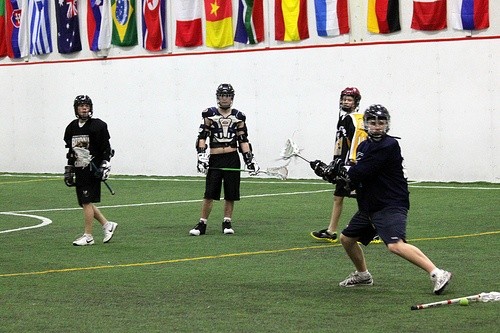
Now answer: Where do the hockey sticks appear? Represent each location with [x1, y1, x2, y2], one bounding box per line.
[73, 147, 115, 195]
[411, 291, 500, 310]
[208, 166, 289, 181]
[280, 139, 311, 164]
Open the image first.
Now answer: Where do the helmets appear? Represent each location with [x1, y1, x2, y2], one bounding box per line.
[339, 87, 361, 112]
[216, 84, 234, 110]
[73, 95, 94, 120]
[363, 104, 390, 139]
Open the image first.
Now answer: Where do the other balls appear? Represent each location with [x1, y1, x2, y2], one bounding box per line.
[459, 298, 468, 306]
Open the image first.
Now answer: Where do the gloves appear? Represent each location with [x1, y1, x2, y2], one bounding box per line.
[324, 163, 347, 186]
[243, 152, 260, 176]
[310, 160, 328, 177]
[64, 165, 75, 187]
[98, 160, 111, 181]
[197, 152, 210, 174]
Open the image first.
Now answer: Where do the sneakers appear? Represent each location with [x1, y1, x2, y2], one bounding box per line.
[339, 272, 373, 287]
[73, 234, 94, 246]
[370, 235, 384, 242]
[311, 228, 339, 242]
[433, 269, 452, 295]
[222, 219, 235, 234]
[103, 221, 118, 243]
[189, 220, 207, 236]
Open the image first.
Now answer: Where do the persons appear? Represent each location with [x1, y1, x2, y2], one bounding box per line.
[311, 87, 381, 244]
[309, 103, 453, 295]
[63, 95, 118, 246]
[189, 84, 260, 236]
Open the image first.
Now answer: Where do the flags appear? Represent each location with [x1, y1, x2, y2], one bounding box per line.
[314, 0, 349, 36]
[175, 0, 202, 47]
[235, 0, 264, 44]
[87, 0, 110, 51]
[273, 0, 309, 41]
[204, 0, 234, 47]
[411, 0, 447, 30]
[111, 0, 138, 47]
[367, 0, 401, 34]
[0, 0, 53, 58]
[55, 0, 82, 54]
[141, 0, 166, 51]
[451, 0, 490, 30]
